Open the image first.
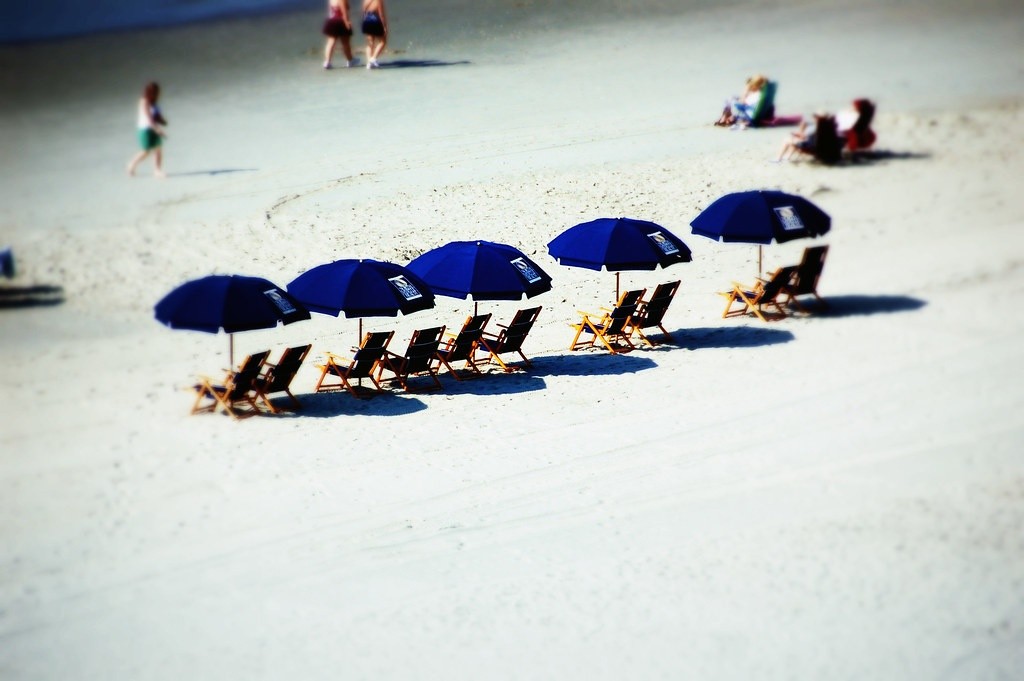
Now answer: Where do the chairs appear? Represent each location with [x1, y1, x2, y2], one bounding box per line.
[224, 343, 314, 415]
[313, 331, 395, 400]
[415, 313, 492, 381]
[618, 279, 682, 349]
[568, 289, 647, 354]
[729, 81, 778, 129]
[192, 350, 275, 419]
[376, 324, 447, 394]
[753, 244, 829, 315]
[464, 305, 542, 373]
[716, 263, 800, 324]
[786, 104, 876, 165]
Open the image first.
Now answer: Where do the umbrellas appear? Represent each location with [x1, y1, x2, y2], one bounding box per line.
[689, 188, 831, 310]
[285, 259, 436, 394]
[546, 218, 693, 353]
[153, 272, 311, 413]
[403, 240, 554, 373]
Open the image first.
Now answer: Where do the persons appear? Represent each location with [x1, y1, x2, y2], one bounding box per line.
[791, 99, 873, 138]
[321, 0, 360, 69]
[769, 110, 831, 161]
[126, 80, 169, 179]
[714, 76, 769, 126]
[361, 0, 388, 69]
